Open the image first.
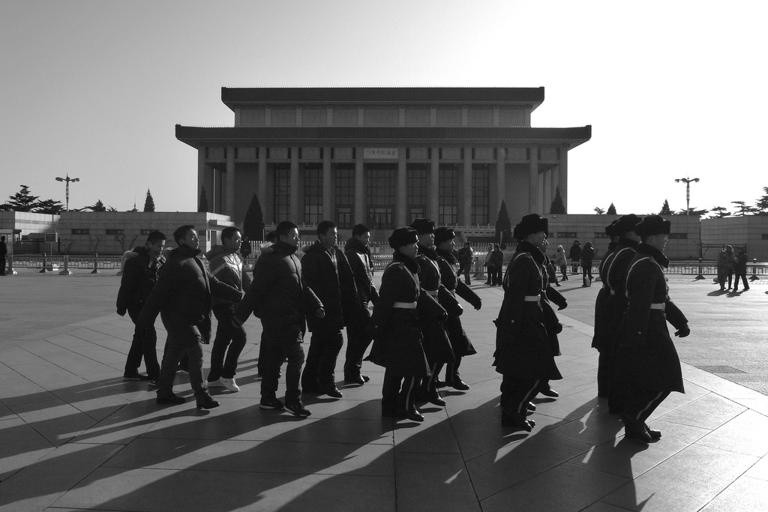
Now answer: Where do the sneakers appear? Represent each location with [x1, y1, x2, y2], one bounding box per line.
[123, 372, 370, 416]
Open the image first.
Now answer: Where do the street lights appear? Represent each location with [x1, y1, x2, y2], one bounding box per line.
[677, 177, 700, 218]
[54, 175, 84, 215]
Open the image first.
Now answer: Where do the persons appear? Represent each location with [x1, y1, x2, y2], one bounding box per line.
[483, 242, 503, 287]
[553, 240, 595, 283]
[717, 244, 734, 290]
[731, 247, 749, 294]
[0, 235, 9, 276]
[591, 214, 689, 443]
[492, 213, 568, 431]
[116, 210, 482, 421]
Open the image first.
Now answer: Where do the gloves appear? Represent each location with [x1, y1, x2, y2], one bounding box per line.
[673, 324, 689, 337]
[557, 301, 568, 309]
[439, 299, 482, 320]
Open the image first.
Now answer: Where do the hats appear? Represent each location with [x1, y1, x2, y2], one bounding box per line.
[514, 214, 548, 241]
[389, 219, 456, 249]
[606, 213, 670, 237]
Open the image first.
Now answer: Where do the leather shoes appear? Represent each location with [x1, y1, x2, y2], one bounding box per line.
[383, 380, 662, 440]
